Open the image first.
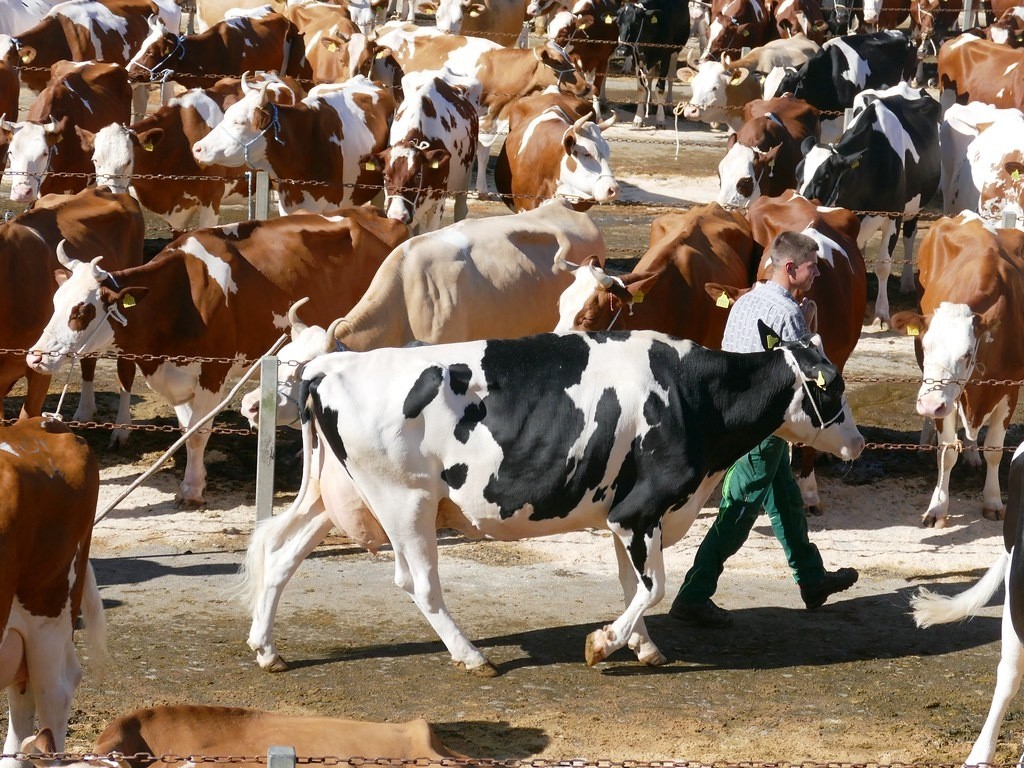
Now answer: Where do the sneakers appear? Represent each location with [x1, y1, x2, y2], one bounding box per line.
[669, 598, 734, 629]
[801, 567, 858, 609]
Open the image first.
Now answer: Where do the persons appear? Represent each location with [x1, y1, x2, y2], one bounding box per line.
[669, 231, 859, 623]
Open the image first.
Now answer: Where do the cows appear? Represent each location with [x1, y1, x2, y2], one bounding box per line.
[899, 437, 1024, 768]
[216, 313, 869, 680]
[12, 701, 492, 768]
[0, 409, 115, 767]
[0, 0, 1024, 530]
